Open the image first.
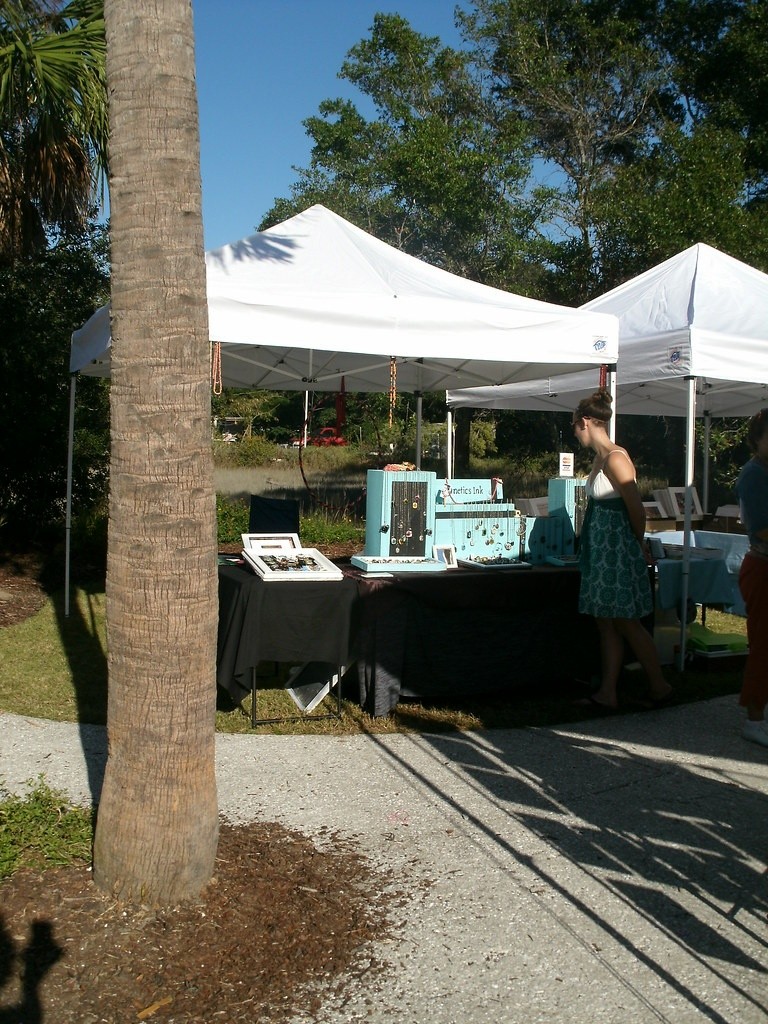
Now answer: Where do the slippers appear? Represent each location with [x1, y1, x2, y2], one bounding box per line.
[572, 697, 617, 713]
[638, 687, 677, 711]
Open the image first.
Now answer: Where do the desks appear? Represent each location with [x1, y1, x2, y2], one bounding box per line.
[645, 515, 751, 629]
[218, 552, 655, 728]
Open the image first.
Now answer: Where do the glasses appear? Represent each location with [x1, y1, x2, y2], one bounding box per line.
[569, 417, 591, 431]
[754, 408, 768, 426]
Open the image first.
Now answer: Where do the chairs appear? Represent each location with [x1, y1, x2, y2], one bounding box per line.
[249, 493, 300, 533]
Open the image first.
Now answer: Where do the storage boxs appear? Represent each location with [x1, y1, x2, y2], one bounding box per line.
[365, 469, 588, 564]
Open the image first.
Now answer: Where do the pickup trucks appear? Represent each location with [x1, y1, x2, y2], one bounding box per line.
[288, 426, 349, 447]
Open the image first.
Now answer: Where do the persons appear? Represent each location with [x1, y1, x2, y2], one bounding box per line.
[735, 408, 768, 746]
[572, 388, 675, 709]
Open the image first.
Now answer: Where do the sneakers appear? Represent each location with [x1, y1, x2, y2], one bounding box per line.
[741, 719, 768, 748]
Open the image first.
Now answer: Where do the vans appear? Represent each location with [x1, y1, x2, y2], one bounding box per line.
[208, 416, 253, 442]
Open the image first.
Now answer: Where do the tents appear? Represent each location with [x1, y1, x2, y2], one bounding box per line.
[447, 241, 767, 673]
[64, 205, 618, 617]
